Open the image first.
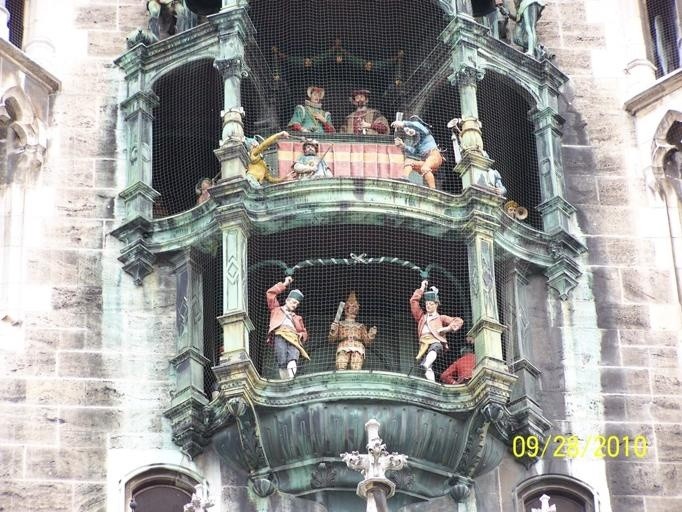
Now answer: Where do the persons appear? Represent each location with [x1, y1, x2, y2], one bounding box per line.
[265, 276, 309, 381]
[292, 138, 334, 180]
[196, 177, 214, 207]
[515, 0, 548, 60]
[288, 86, 336, 133]
[409, 279, 464, 381]
[502, 201, 519, 217]
[145, 1, 186, 44]
[341, 89, 389, 136]
[391, 120, 442, 190]
[328, 292, 376, 371]
[244, 131, 298, 189]
